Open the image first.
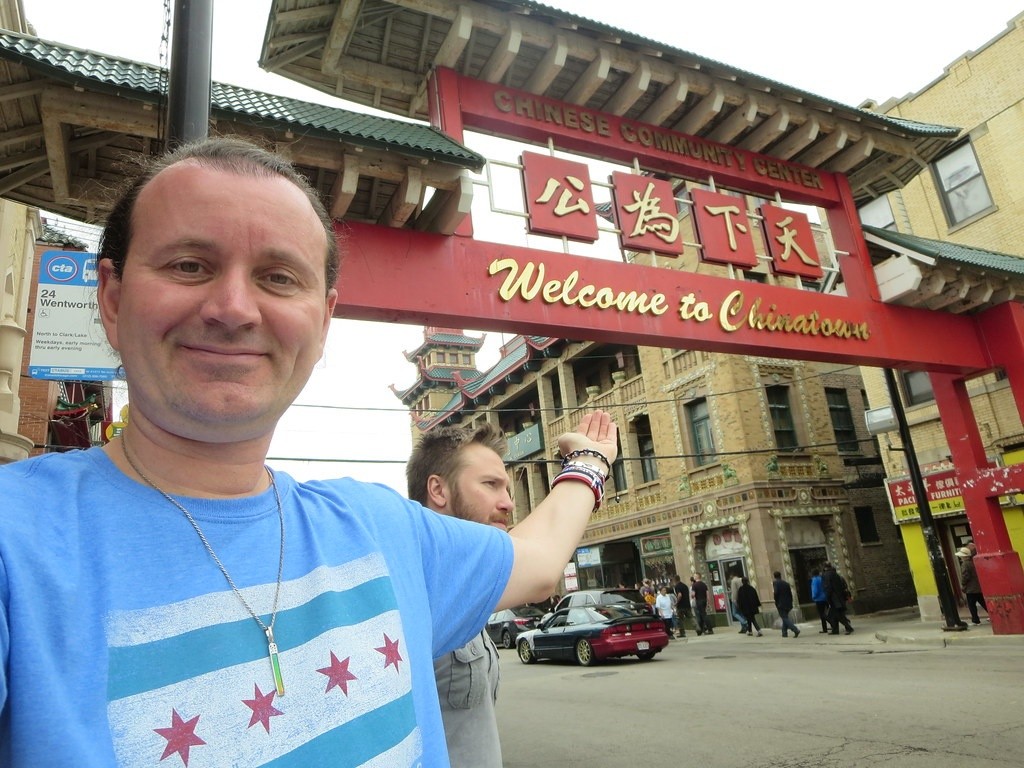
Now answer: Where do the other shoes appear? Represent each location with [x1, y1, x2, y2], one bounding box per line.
[794, 630, 800, 638]
[828, 631, 840, 635]
[819, 630, 827, 633]
[677, 633, 686, 637]
[844, 629, 854, 635]
[756, 631, 763, 637]
[973, 622, 981, 626]
[747, 632, 753, 636]
[697, 631, 702, 635]
[706, 629, 713, 634]
[739, 629, 746, 633]
[782, 634, 788, 637]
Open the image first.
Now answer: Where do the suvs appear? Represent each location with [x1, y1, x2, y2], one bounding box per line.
[540, 587, 653, 627]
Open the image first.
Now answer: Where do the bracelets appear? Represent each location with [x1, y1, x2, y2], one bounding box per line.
[550, 449, 611, 514]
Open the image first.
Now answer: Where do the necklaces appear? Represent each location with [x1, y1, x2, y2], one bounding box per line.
[119, 429, 287, 697]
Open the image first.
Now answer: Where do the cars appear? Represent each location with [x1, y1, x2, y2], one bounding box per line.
[515, 604, 669, 666]
[484, 606, 546, 649]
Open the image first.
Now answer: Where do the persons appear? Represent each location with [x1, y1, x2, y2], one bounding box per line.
[639, 571, 714, 639]
[1, 137, 619, 768]
[773, 570, 800, 638]
[961, 540, 991, 626]
[727, 567, 763, 638]
[404, 425, 515, 768]
[810, 562, 855, 635]
[551, 595, 560, 614]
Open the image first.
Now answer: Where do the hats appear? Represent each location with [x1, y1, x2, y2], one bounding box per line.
[955, 547, 971, 557]
